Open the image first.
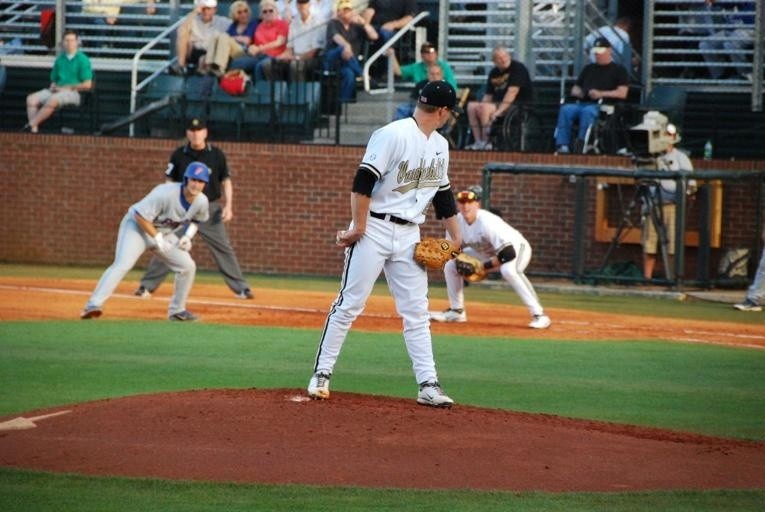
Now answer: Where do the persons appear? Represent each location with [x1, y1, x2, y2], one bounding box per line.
[230, 0, 288, 81]
[640, 124, 696, 281]
[733, 246, 765, 311]
[555, 37, 629, 156]
[197, 0, 259, 78]
[307, 81, 462, 409]
[134, 117, 255, 300]
[262, 1, 326, 80]
[322, 0, 378, 103]
[369, 0, 421, 47]
[582, 15, 631, 77]
[80, 162, 210, 322]
[385, 43, 458, 123]
[168, 0, 233, 78]
[464, 47, 533, 152]
[20, 30, 92, 134]
[678, 2, 754, 80]
[330, 0, 369, 22]
[430, 185, 552, 329]
[410, 65, 456, 151]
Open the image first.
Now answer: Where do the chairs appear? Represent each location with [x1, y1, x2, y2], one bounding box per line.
[144, 74, 182, 131]
[281, 81, 321, 140]
[169, 75, 218, 131]
[208, 77, 248, 138]
[253, 81, 286, 138]
[59, 77, 100, 128]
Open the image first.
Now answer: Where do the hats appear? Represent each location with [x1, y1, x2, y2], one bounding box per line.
[186, 116, 206, 130]
[199, 0, 218, 8]
[336, 0, 352, 11]
[589, 36, 612, 54]
[418, 79, 465, 114]
[420, 41, 435, 49]
[456, 190, 476, 203]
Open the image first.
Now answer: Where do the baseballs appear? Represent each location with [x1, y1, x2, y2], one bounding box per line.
[335, 230, 346, 241]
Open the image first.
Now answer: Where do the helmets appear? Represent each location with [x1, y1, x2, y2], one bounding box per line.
[183, 160, 210, 186]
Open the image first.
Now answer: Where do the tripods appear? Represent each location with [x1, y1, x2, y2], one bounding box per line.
[593, 181, 675, 289]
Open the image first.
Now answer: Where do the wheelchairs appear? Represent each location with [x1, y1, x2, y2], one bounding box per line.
[465, 104, 545, 152]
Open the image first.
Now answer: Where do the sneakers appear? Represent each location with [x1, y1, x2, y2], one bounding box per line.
[196, 67, 205, 75]
[429, 309, 467, 323]
[209, 68, 222, 77]
[168, 63, 188, 76]
[79, 306, 103, 319]
[733, 298, 764, 313]
[528, 315, 553, 330]
[134, 284, 152, 297]
[416, 382, 455, 408]
[234, 287, 255, 299]
[471, 140, 493, 150]
[307, 373, 330, 399]
[464, 140, 481, 150]
[168, 310, 200, 322]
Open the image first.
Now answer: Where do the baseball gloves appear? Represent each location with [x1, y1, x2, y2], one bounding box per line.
[416, 236, 461, 269]
[452, 253, 485, 282]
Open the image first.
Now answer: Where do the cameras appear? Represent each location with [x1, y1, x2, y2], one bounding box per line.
[627, 109, 671, 169]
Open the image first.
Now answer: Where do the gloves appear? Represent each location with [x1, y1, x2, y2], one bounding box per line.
[178, 221, 198, 251]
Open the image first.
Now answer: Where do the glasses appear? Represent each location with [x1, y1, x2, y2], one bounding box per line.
[263, 8, 274, 14]
[423, 48, 435, 53]
[237, 8, 249, 14]
